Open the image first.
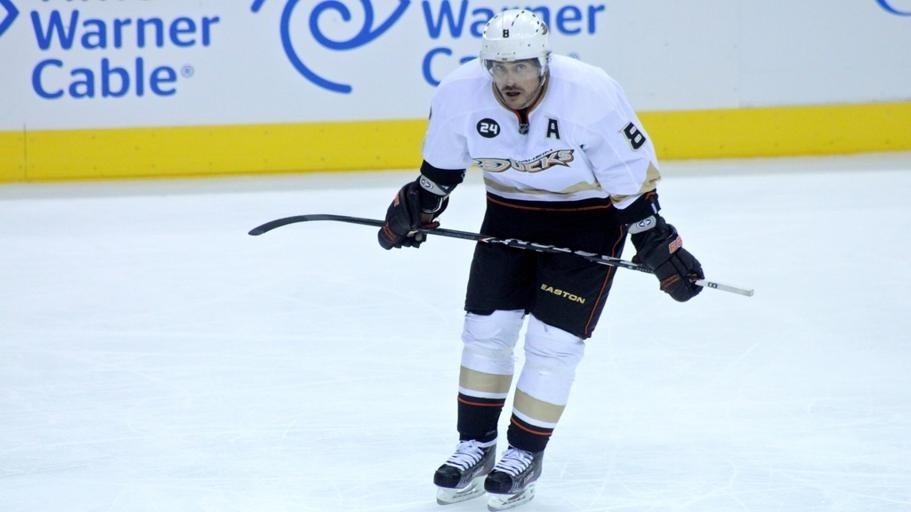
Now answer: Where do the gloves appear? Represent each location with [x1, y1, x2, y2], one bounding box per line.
[377, 175, 450, 250]
[631, 224, 705, 302]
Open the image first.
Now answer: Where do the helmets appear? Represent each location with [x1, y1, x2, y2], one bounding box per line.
[478, 9, 552, 77]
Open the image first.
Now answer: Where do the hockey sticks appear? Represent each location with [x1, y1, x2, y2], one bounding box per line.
[248, 214, 753, 298]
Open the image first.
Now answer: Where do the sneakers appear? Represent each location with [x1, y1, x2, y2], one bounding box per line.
[433, 438, 497, 490]
[484, 446, 544, 495]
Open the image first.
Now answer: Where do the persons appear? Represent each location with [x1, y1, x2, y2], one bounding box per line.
[378, 9, 704, 492]
